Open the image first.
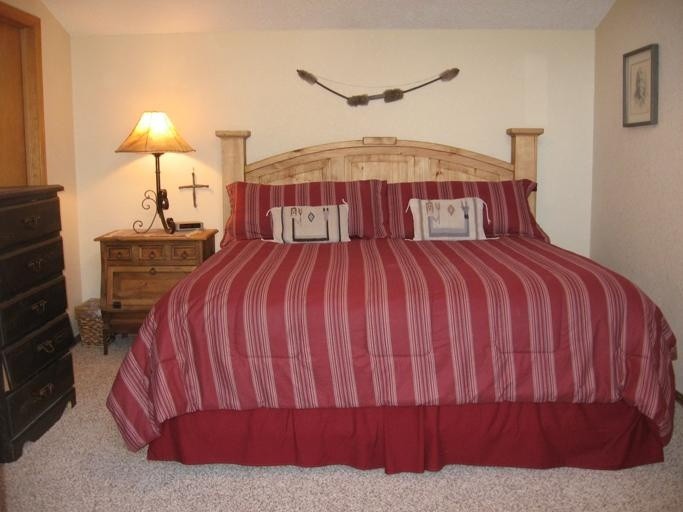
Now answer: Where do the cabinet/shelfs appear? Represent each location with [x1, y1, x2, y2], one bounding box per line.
[0, 184, 76, 466]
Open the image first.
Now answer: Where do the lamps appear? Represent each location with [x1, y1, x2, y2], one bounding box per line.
[116, 112, 196, 234]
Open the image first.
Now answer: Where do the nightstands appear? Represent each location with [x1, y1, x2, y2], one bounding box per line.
[94, 228, 219, 355]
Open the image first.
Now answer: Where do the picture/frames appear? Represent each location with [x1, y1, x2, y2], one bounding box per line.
[623, 43, 658, 128]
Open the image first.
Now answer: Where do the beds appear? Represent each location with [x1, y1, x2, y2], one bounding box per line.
[148, 129, 678, 474]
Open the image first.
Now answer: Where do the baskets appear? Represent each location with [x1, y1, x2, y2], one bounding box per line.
[73, 297, 115, 348]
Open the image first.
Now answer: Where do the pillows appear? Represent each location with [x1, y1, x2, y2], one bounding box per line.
[405, 197, 492, 242]
[261, 199, 351, 244]
[387, 180, 548, 242]
[221, 180, 391, 246]
